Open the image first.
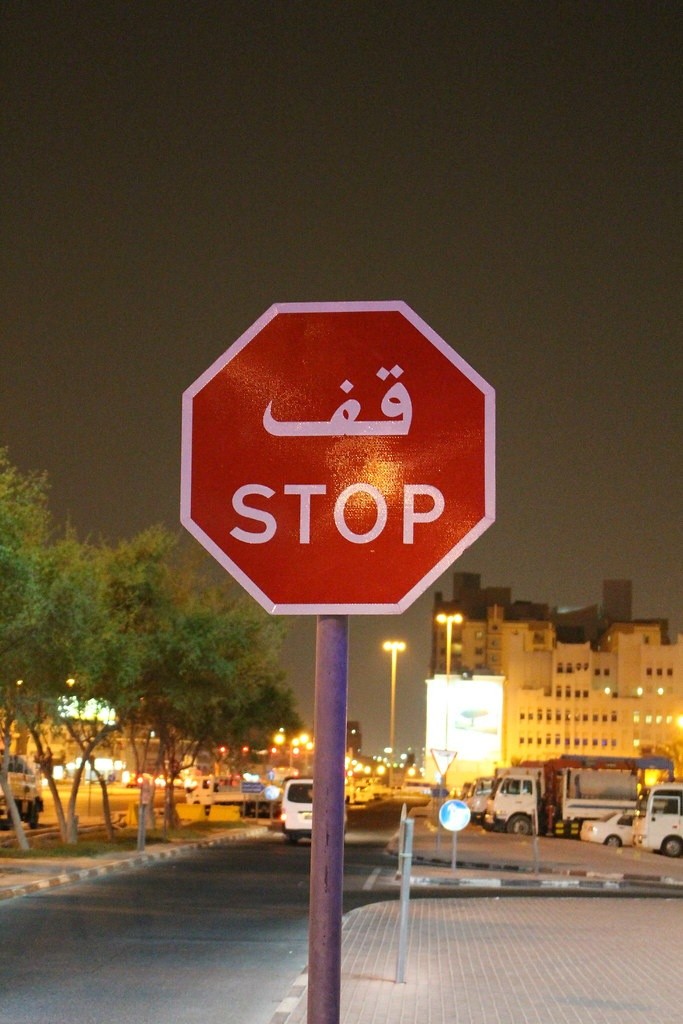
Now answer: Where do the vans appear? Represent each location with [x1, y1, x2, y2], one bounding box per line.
[631, 782, 683, 858]
[280, 779, 348, 845]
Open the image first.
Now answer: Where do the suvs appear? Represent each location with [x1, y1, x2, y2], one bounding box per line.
[458, 775, 494, 823]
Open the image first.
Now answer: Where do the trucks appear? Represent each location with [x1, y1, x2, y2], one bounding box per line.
[0, 756, 43, 830]
[484, 766, 641, 835]
[185, 775, 284, 817]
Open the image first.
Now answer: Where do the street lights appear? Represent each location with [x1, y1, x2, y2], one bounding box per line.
[383, 639, 408, 789]
[436, 613, 464, 754]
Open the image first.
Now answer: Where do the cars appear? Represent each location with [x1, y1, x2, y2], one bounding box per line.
[579, 810, 636, 850]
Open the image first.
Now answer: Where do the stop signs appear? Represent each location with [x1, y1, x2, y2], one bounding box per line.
[178, 299, 498, 614]
